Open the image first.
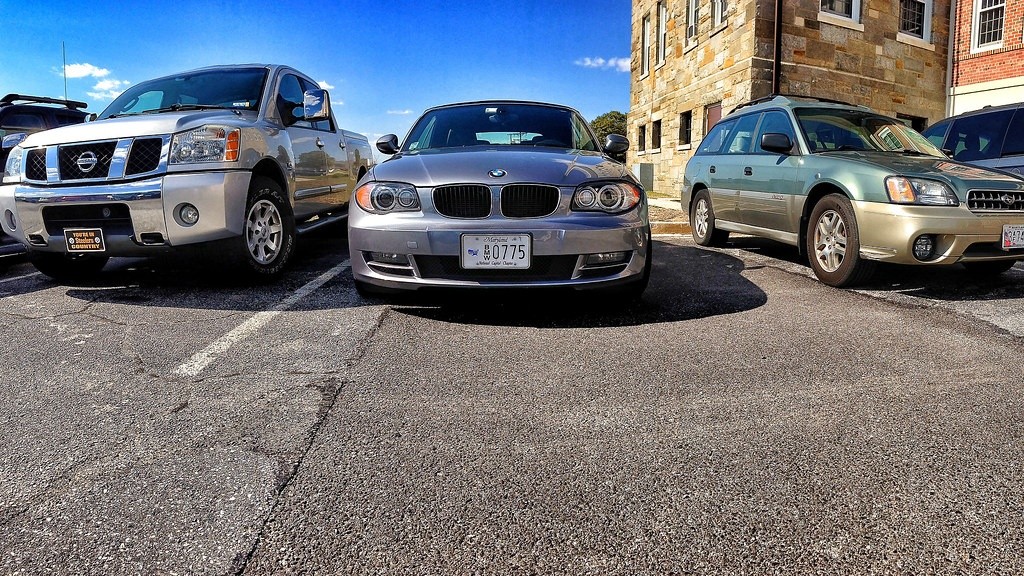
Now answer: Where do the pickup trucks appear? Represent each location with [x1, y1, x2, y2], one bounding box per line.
[0, 61, 377, 284]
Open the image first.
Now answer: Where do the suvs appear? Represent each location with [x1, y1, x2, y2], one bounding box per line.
[679, 92, 1024, 291]
[0, 92, 91, 256]
[917, 102, 1024, 177]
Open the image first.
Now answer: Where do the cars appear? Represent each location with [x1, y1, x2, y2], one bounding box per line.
[348, 98, 655, 297]
